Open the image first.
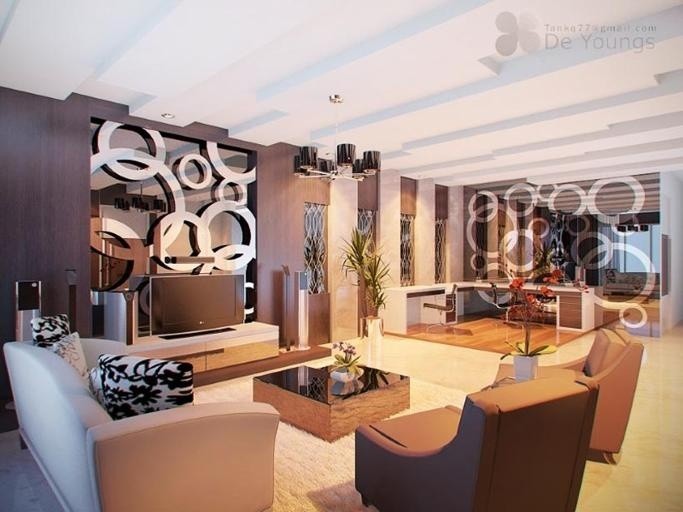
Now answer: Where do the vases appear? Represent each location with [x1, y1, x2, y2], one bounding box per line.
[330, 365, 365, 383]
[513, 355, 538, 383]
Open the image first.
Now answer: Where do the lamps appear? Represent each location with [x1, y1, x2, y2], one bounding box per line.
[617, 213, 649, 232]
[292, 94, 382, 184]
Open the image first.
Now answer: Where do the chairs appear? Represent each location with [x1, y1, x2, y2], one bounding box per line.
[354, 378, 600, 512]
[492, 324, 644, 466]
[424, 284, 458, 335]
[490, 281, 526, 322]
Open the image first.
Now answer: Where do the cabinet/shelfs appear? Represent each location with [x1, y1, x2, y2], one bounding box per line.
[128, 319, 280, 375]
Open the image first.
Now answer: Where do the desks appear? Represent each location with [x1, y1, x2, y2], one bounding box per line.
[400, 281, 603, 333]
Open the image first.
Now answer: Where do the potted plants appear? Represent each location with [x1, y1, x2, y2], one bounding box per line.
[335, 226, 392, 338]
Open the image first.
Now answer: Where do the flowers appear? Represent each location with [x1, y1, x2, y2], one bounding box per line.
[328, 342, 362, 376]
[488, 267, 565, 359]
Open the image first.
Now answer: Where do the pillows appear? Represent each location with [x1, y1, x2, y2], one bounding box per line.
[29, 312, 71, 349]
[53, 331, 88, 381]
[90, 352, 194, 420]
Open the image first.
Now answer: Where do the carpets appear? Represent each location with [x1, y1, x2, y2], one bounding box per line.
[194, 356, 475, 512]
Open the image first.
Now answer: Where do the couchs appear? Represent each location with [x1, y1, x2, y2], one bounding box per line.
[604, 271, 660, 295]
[2, 337, 281, 512]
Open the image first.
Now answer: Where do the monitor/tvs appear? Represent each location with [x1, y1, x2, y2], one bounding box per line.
[148, 274, 246, 340]
